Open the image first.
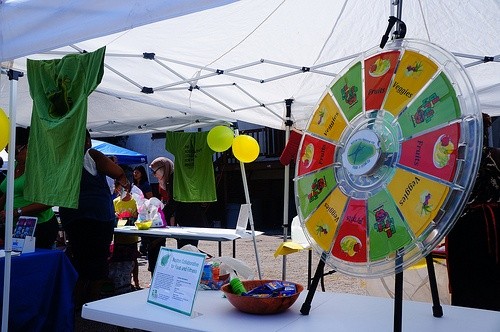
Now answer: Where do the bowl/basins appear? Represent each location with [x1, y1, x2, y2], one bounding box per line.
[221, 279, 304, 314]
[218, 273, 231, 281]
[134, 220, 152, 230]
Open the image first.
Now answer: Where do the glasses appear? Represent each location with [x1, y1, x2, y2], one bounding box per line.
[153, 166, 161, 174]
[5, 144, 25, 154]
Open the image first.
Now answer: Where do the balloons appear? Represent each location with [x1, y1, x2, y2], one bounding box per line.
[207, 126, 260, 163]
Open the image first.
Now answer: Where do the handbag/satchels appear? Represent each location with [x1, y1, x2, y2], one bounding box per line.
[137, 197, 167, 227]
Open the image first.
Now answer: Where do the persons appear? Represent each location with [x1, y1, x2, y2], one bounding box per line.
[433, 113, 500, 311]
[60, 127, 118, 311]
[106, 156, 203, 289]
[0, 126, 59, 252]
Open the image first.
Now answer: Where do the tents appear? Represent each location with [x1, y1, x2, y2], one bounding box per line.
[0, 0, 500, 332]
[91, 139, 151, 186]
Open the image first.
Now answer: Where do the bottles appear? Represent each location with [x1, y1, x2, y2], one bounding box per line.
[203, 262, 220, 281]
[230, 278, 247, 296]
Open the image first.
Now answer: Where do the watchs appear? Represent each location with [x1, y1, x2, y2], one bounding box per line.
[17, 208, 22, 216]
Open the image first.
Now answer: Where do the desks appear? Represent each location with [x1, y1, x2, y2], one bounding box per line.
[80, 289, 500, 332]
[113, 227, 262, 275]
[0, 249, 77, 332]
